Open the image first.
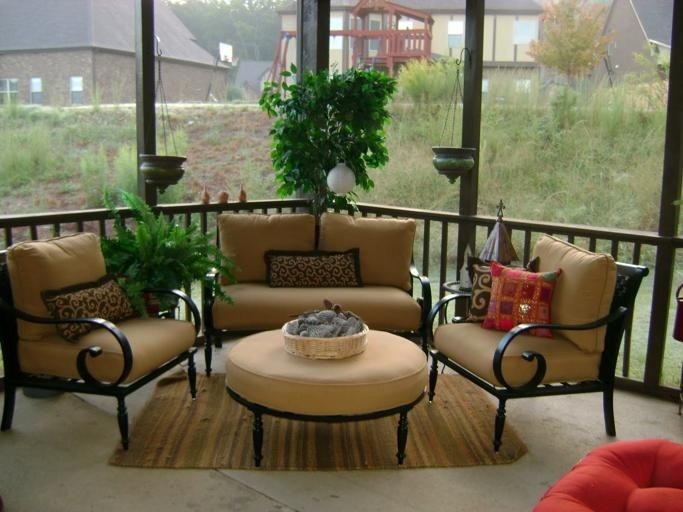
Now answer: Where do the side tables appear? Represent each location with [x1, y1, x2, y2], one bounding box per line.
[443, 279, 474, 376]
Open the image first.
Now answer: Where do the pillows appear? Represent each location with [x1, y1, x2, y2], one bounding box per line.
[40, 275, 143, 344]
[464, 255, 562, 338]
[264, 248, 363, 288]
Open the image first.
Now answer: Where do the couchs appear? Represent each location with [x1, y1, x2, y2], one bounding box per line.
[202, 211, 432, 378]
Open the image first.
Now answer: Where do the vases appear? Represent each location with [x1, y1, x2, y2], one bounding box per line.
[432, 146, 476, 183]
[138, 153, 188, 194]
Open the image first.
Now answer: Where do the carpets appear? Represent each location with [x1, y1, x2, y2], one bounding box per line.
[107, 369, 528, 474]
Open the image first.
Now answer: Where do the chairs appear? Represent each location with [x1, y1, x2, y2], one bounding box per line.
[0, 230, 203, 452]
[428, 234, 650, 454]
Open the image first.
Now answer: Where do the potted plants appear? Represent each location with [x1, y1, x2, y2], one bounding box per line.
[96, 186, 242, 319]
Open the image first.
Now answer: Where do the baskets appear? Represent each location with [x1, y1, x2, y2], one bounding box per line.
[283, 319, 369, 359]
